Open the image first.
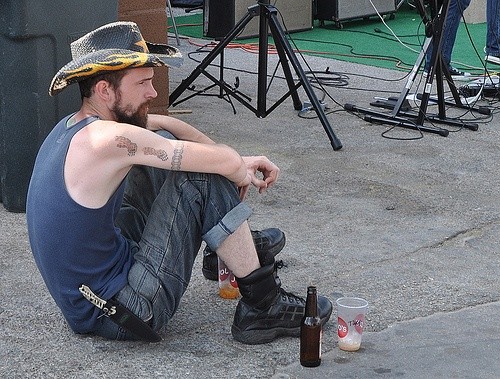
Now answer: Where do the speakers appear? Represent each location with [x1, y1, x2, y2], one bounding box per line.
[203, 1, 315, 41]
[314, 0, 397, 24]
[0, 0, 118, 213]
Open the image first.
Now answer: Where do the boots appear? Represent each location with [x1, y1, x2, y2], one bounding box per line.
[231, 249, 332, 345]
[202, 222, 285, 283]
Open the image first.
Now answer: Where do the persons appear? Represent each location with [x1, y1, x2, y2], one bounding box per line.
[422, 0, 500, 79]
[26, 20, 332, 345]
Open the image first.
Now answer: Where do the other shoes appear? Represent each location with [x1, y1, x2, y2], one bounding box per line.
[422, 63, 471, 80]
[484, 54, 499, 64]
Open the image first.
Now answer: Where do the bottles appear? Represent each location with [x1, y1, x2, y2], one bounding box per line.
[300, 285, 322, 368]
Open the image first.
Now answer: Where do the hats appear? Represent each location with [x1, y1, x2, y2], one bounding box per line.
[48, 18, 184, 97]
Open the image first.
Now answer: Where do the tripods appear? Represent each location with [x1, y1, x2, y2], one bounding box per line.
[167, 0, 343, 151]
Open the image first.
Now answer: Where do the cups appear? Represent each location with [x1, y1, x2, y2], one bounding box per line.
[335, 297, 368, 352]
[218, 255, 239, 299]
